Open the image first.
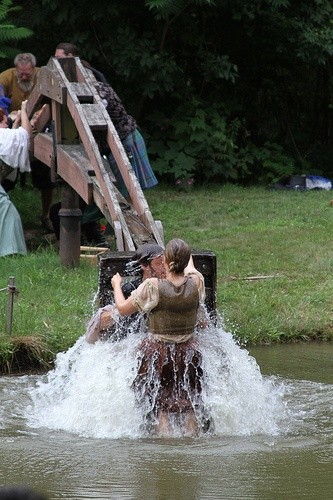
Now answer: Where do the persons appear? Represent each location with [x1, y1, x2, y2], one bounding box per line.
[88, 243, 210, 344]
[110, 237, 214, 437]
[0, 98, 32, 256]
[0, 53, 56, 232]
[55, 42, 157, 208]
